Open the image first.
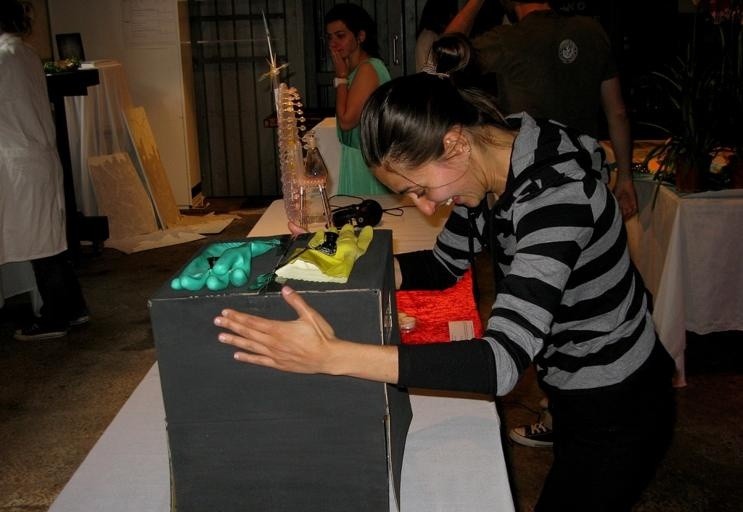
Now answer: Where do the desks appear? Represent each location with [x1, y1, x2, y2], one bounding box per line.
[302, 115, 344, 193]
[40, 70, 109, 257]
[48, 194, 518, 512]
[601, 136, 743, 386]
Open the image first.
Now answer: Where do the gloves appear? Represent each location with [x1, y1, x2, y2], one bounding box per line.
[276, 224, 374, 284]
[171, 239, 281, 293]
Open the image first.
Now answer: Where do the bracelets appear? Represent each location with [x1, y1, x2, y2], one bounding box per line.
[333, 77, 347, 88]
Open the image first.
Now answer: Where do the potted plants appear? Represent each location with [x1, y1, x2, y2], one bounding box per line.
[642, 27, 730, 198]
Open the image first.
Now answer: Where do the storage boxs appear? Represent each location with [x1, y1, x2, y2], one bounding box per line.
[144, 223, 412, 510]
[15, 306, 87, 363]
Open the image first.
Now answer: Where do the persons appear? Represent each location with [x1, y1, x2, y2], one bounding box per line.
[0, 1, 90, 342]
[323, 4, 392, 196]
[214, 31, 677, 512]
[415, 0, 501, 97]
[441, 0, 640, 447]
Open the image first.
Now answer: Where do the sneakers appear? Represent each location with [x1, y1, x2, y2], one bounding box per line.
[510, 423, 553, 449]
[13, 309, 88, 341]
